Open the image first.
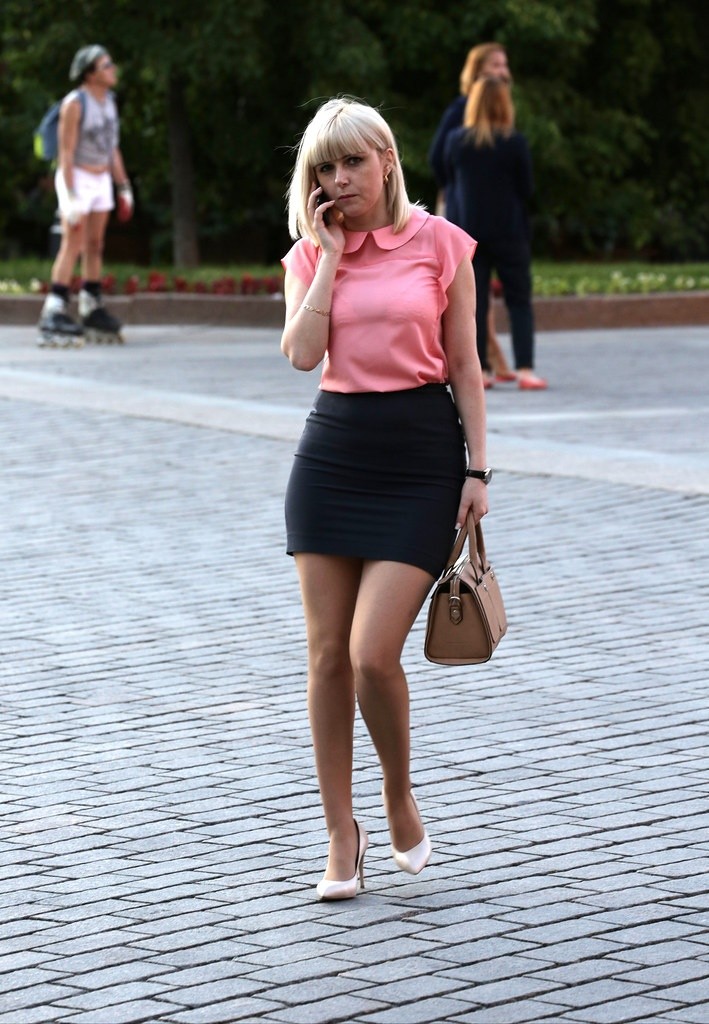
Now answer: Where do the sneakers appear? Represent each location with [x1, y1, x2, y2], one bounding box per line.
[76, 289, 125, 345]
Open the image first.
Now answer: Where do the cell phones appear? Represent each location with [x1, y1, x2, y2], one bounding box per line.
[317, 184, 329, 227]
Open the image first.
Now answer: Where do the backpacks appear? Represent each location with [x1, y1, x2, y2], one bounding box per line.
[33, 90, 86, 161]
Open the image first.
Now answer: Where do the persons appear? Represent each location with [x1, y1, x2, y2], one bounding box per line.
[431, 42, 549, 390]
[35, 44, 133, 347]
[279, 96, 484, 902]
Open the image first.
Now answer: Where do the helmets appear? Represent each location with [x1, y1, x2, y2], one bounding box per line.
[69, 44, 106, 81]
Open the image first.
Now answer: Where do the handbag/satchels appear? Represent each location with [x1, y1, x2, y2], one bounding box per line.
[424, 508, 507, 665]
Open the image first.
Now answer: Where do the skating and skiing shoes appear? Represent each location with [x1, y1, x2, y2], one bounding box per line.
[35, 292, 85, 348]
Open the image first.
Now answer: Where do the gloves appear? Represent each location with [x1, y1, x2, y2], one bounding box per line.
[118, 189, 133, 220]
[67, 201, 82, 232]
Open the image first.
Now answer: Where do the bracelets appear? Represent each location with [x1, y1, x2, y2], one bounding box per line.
[303, 305, 331, 317]
[117, 181, 131, 189]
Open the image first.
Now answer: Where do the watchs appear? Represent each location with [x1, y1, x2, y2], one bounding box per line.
[465, 469, 492, 484]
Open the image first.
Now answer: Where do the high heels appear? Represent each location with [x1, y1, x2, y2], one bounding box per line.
[381, 790, 431, 874]
[316, 818, 369, 899]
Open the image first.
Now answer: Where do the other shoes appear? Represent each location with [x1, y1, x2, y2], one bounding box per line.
[493, 370, 516, 382]
[482, 376, 493, 388]
[518, 377, 546, 390]
